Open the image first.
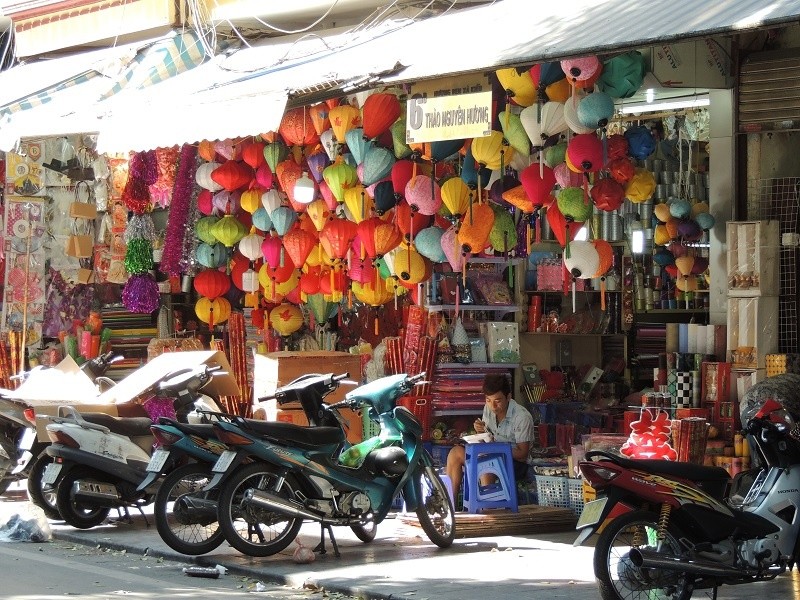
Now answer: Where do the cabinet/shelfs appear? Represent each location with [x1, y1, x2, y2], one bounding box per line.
[425, 258, 521, 416]
[524, 240, 625, 337]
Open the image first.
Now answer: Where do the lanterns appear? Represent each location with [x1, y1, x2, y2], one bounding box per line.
[193, 45, 716, 353]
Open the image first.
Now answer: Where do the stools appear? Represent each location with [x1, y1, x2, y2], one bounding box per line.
[403, 475, 455, 516]
[462, 442, 518, 513]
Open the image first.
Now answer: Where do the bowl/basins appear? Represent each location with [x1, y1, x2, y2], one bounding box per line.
[462, 432, 491, 444]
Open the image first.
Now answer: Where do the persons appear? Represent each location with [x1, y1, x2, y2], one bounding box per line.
[446, 374, 534, 511]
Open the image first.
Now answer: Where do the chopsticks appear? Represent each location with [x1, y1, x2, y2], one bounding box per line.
[478, 418, 490, 433]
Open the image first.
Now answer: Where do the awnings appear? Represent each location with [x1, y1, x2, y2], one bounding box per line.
[0, 27, 214, 138]
[96, 0, 800, 158]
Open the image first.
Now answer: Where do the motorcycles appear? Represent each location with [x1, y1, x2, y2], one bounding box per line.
[196, 370, 456, 559]
[135, 371, 377, 556]
[573, 398, 800, 600]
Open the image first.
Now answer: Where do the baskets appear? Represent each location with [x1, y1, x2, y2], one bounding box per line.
[568, 478, 586, 517]
[534, 473, 569, 508]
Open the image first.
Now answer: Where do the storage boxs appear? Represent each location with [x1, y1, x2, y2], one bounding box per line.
[701, 220, 780, 444]
[527, 401, 584, 425]
[253, 351, 363, 443]
[362, 395, 452, 466]
[534, 475, 584, 515]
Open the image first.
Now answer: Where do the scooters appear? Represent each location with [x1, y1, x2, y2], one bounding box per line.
[0, 351, 231, 530]
